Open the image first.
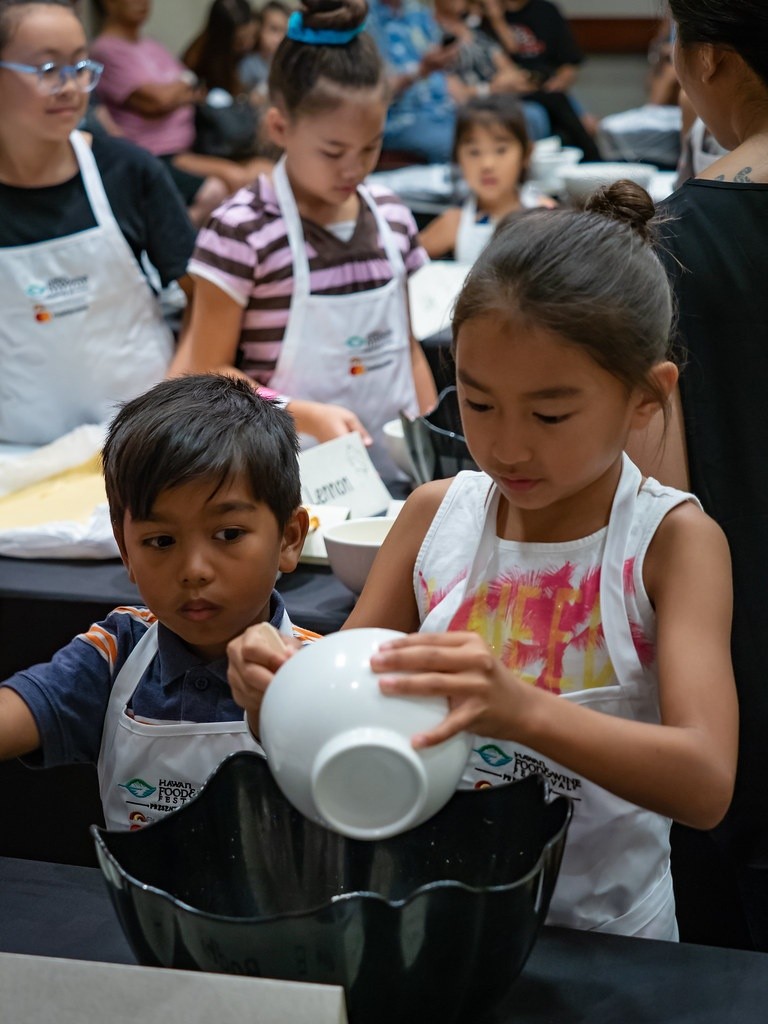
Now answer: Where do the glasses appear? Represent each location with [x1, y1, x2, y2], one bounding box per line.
[0, 59, 104, 97]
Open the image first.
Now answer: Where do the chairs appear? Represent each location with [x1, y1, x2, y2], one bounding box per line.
[400, 385, 474, 483]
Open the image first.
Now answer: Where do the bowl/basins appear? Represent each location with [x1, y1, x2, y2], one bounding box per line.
[88, 750, 575, 1024]
[323, 516, 397, 597]
[565, 159, 658, 206]
[525, 148, 585, 185]
[258, 627, 476, 841]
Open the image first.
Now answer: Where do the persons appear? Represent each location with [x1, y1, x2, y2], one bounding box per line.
[0, 0, 197, 447]
[659, 0, 768, 949]
[168, 0, 437, 484]
[647, 18, 729, 190]
[0, 375, 325, 833]
[172, 0, 307, 195]
[410, 95, 560, 263]
[90, 0, 225, 225]
[363, 0, 604, 163]
[225, 182, 739, 938]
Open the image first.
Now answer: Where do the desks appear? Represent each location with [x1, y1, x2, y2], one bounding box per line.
[0, 858, 768, 1024]
[0, 548, 355, 632]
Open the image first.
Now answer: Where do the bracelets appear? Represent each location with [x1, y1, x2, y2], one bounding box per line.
[254, 386, 292, 410]
[477, 83, 490, 99]
[403, 61, 421, 82]
